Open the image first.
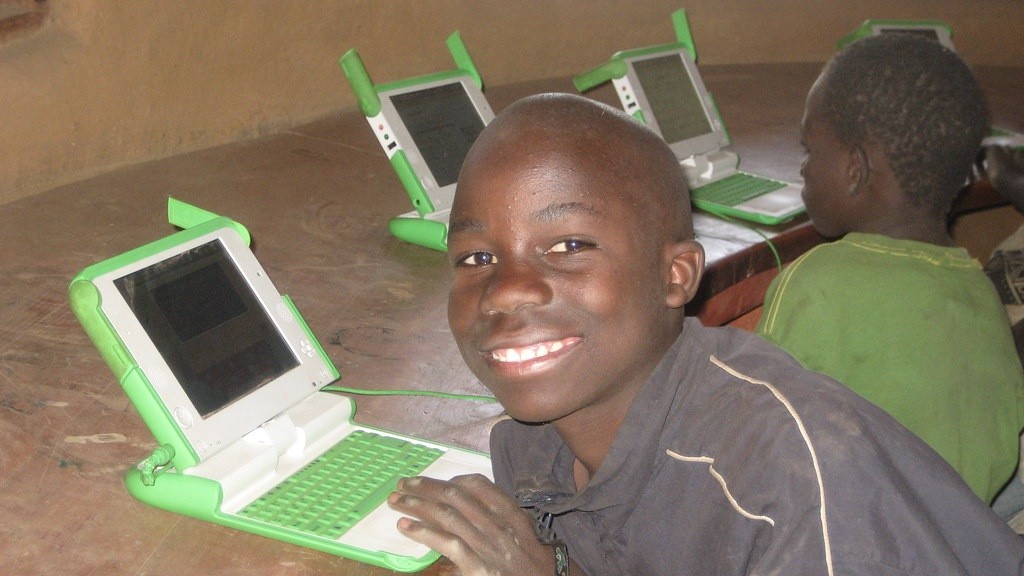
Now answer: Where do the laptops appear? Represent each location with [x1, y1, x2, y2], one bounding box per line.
[69, 198, 497, 574]
[839, 18, 1024, 153]
[339, 30, 496, 252]
[573, 6, 808, 224]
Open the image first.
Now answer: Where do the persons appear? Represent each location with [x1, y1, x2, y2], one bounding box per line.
[753, 33, 1024, 508]
[974, 141, 1024, 213]
[387, 92, 1024, 576]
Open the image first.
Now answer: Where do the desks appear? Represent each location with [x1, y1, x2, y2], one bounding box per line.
[0, 58, 1024, 576]
